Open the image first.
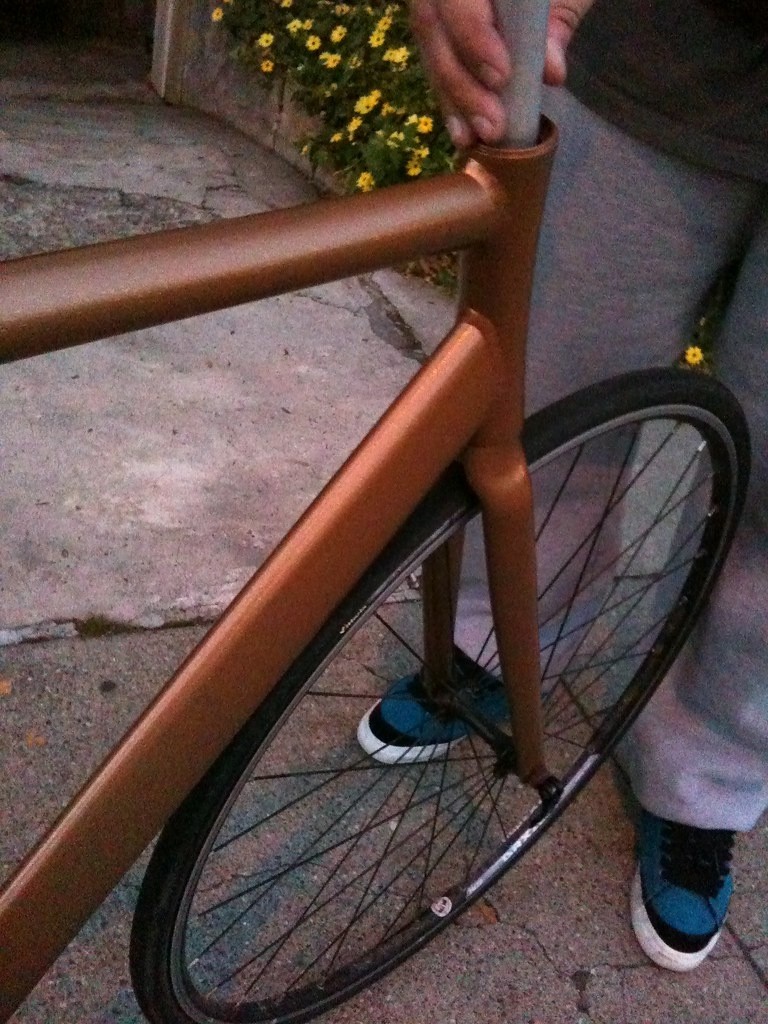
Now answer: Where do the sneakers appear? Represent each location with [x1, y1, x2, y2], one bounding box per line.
[631, 807, 734, 975]
[357, 655, 512, 765]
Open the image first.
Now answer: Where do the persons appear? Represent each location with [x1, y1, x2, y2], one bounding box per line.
[357, 0, 768, 973]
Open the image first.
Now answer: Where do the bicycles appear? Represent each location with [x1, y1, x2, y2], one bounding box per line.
[0, 0, 752, 1023]
